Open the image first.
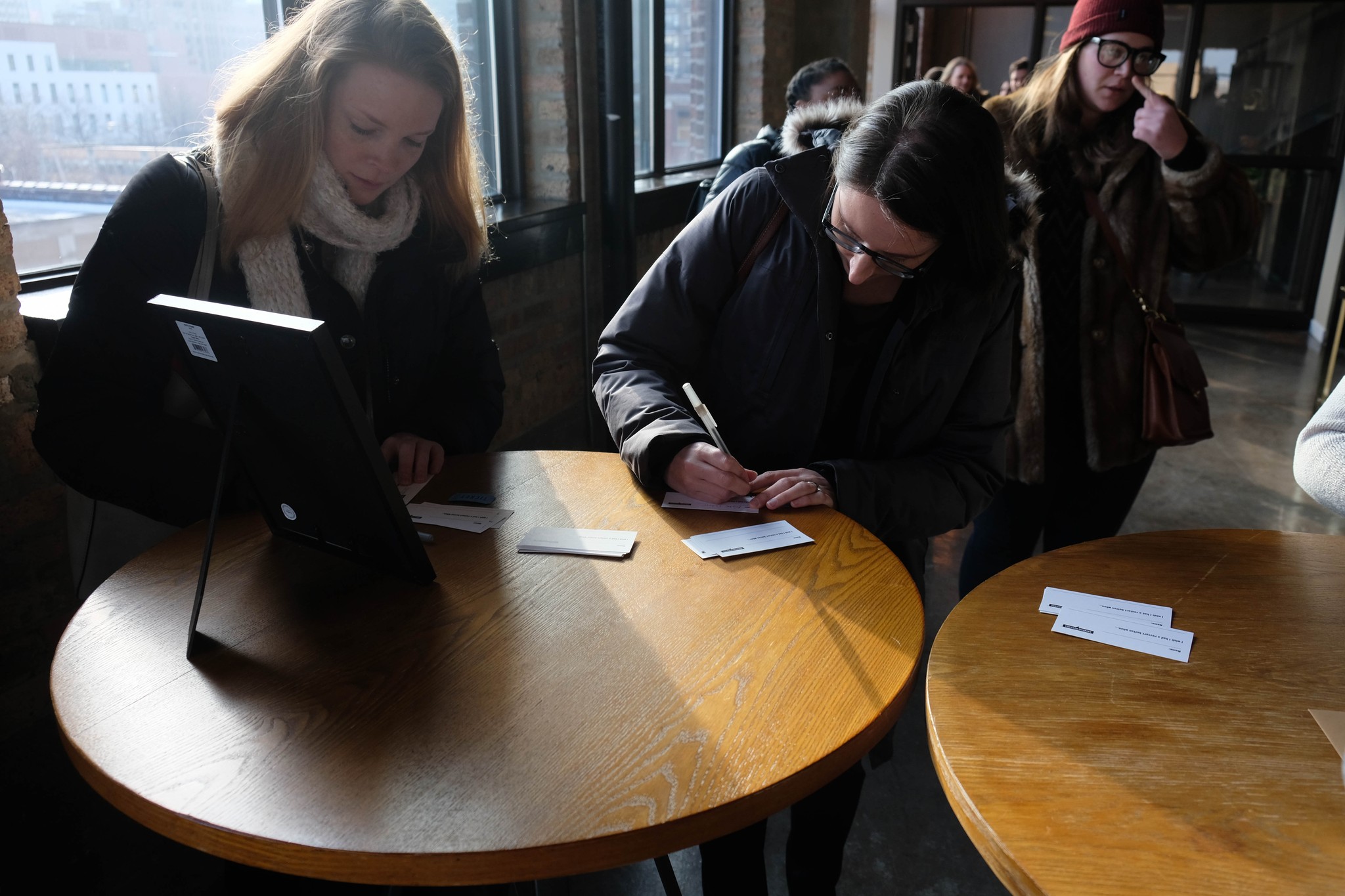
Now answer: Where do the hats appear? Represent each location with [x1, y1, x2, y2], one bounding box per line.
[1060, 0, 1166, 56]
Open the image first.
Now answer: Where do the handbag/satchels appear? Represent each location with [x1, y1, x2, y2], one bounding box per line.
[1138, 311, 1219, 457]
[59, 471, 174, 607]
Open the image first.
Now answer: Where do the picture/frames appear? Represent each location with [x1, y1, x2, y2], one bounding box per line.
[145, 291, 436, 650]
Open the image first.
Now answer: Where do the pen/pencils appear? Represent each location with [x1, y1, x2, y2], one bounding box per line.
[682, 382, 754, 498]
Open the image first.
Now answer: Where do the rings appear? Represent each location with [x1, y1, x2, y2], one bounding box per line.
[807, 481, 823, 493]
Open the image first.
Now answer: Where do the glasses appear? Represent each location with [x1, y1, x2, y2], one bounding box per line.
[811, 87, 864, 104]
[823, 179, 945, 280]
[1087, 33, 1165, 80]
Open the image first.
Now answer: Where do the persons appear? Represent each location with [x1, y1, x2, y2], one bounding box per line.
[587, 78, 1042, 896]
[37, 0, 507, 894]
[957, 0, 1268, 599]
[687, 51, 1057, 224]
[1291, 343, 1345, 514]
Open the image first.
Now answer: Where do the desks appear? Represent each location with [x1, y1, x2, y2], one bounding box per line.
[49, 446, 933, 896]
[901, 525, 1344, 896]
